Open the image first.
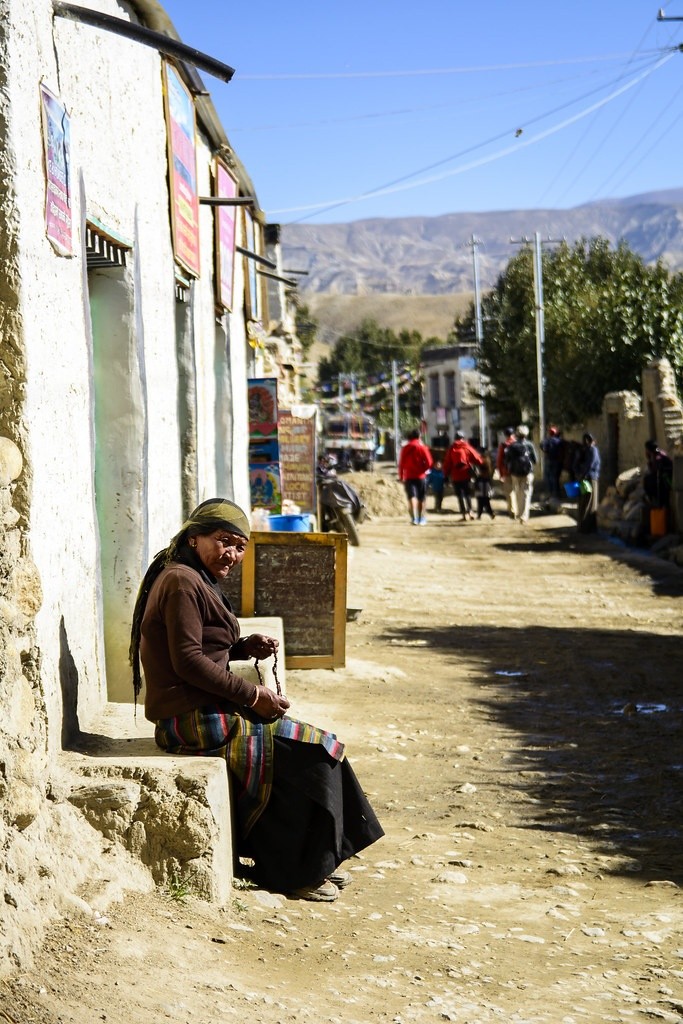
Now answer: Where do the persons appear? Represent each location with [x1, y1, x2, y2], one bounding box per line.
[398, 429, 431, 525]
[426, 462, 450, 511]
[497, 427, 519, 519]
[540, 426, 573, 498]
[469, 439, 495, 520]
[504, 424, 537, 524]
[130, 499, 385, 901]
[442, 432, 449, 447]
[443, 432, 483, 520]
[578, 433, 600, 528]
[643, 441, 673, 539]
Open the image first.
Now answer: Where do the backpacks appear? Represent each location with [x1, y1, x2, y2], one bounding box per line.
[509, 441, 531, 477]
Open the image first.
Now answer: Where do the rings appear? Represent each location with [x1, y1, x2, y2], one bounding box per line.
[271, 714, 277, 718]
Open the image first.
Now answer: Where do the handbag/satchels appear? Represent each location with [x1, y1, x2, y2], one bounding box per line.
[650, 508, 666, 536]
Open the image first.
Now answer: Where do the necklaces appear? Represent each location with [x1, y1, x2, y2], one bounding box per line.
[254, 639, 281, 697]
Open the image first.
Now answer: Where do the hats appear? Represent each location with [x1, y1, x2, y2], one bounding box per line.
[550, 428, 556, 435]
[516, 425, 529, 437]
[455, 432, 464, 438]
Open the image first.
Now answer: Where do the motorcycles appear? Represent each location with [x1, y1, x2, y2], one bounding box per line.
[315, 454, 364, 547]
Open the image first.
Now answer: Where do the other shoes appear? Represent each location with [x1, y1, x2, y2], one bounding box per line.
[411, 517, 426, 526]
[300, 878, 339, 901]
[329, 867, 353, 888]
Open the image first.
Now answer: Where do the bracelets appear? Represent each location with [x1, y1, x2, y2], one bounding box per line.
[245, 685, 259, 708]
